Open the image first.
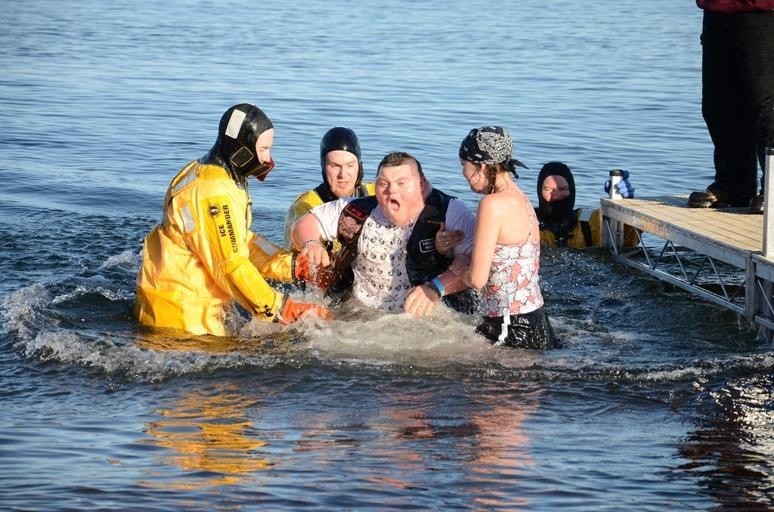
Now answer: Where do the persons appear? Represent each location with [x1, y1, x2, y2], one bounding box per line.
[136, 102, 335, 336]
[286, 127, 376, 257]
[534, 162, 642, 249]
[687, 0, 774, 213]
[290, 153, 476, 316]
[435, 126, 555, 349]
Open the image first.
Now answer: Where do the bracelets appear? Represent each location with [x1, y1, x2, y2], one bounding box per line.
[302, 240, 326, 249]
[426, 279, 445, 298]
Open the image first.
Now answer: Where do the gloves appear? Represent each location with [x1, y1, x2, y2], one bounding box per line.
[273, 295, 332, 326]
[604, 170, 634, 198]
[292, 251, 337, 292]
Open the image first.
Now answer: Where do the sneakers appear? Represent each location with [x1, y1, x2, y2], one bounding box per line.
[686, 186, 764, 214]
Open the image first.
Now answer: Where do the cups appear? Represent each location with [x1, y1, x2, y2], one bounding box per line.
[609, 170, 624, 200]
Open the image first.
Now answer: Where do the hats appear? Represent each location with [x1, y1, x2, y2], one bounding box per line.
[459, 126, 512, 164]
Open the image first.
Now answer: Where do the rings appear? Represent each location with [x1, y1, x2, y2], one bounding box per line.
[447, 241, 449, 245]
